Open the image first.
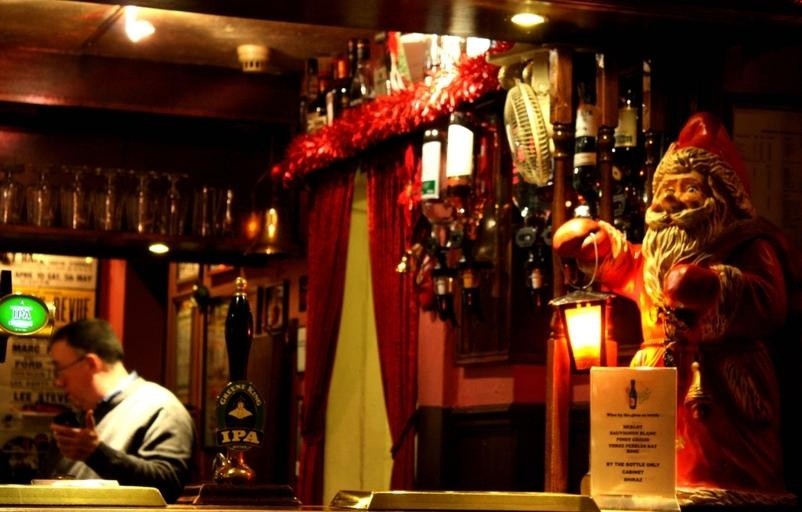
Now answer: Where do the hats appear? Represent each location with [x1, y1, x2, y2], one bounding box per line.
[645, 111, 756, 219]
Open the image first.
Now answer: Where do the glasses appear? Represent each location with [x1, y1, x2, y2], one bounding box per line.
[49, 354, 89, 377]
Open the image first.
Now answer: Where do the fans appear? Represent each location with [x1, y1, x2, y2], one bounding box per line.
[504, 83, 554, 187]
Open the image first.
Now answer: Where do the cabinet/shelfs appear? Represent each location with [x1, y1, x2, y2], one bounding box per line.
[0, 53, 300, 262]
[167, 264, 309, 502]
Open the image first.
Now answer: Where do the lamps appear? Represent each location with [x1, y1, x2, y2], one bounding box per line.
[149, 243, 169, 254]
[548, 288, 617, 376]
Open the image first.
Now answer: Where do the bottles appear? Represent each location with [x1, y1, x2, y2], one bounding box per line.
[445, 103, 474, 194]
[305, 37, 370, 133]
[420, 120, 445, 201]
[612, 88, 641, 154]
[630, 380, 636, 409]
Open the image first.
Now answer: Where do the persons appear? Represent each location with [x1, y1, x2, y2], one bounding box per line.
[45, 317, 199, 499]
[551, 111, 790, 494]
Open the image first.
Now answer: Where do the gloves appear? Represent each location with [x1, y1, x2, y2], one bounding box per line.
[551, 217, 629, 274]
[666, 261, 742, 344]
